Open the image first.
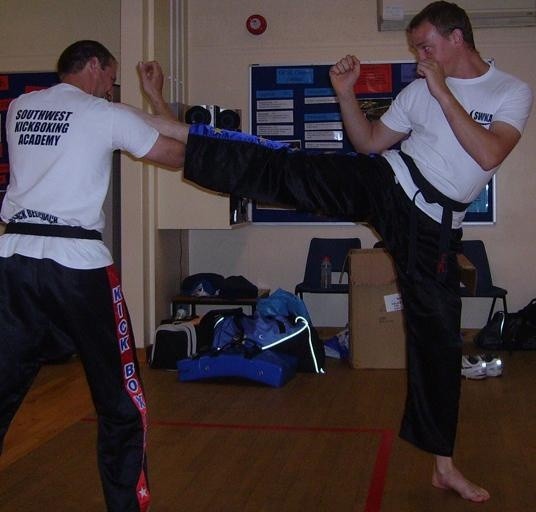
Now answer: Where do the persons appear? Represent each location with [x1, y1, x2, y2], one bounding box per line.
[0, 40, 187, 512]
[116, 1, 533, 502]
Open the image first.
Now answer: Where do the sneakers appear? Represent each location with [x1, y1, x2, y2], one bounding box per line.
[461, 354, 487, 381]
[481, 352, 503, 377]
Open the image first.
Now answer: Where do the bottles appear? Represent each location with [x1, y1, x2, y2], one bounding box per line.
[320, 255, 332, 290]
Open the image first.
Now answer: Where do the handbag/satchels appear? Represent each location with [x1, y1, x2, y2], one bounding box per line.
[145, 314, 203, 373]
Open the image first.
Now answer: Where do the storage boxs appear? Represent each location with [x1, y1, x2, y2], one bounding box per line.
[347, 244, 405, 372]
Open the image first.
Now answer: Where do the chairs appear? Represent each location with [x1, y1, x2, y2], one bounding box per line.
[294, 238, 510, 351]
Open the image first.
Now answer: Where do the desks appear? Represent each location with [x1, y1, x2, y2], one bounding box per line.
[172, 289, 271, 320]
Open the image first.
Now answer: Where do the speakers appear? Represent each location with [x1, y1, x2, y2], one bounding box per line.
[219, 108, 242, 132]
[183, 103, 220, 128]
